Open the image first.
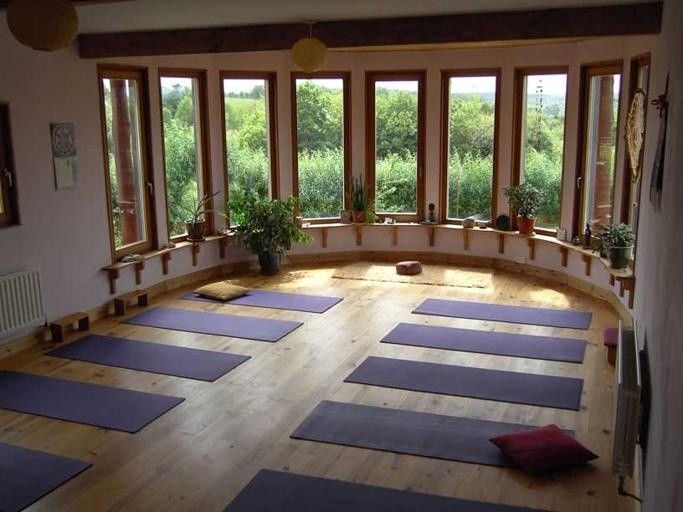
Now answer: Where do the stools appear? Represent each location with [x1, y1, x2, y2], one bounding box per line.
[604, 327, 617, 365]
[113, 289, 153, 313]
[49, 311, 92, 342]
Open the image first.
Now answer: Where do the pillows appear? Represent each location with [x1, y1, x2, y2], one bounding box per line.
[194, 281, 249, 303]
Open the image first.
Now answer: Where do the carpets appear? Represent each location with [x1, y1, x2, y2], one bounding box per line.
[333, 259, 499, 289]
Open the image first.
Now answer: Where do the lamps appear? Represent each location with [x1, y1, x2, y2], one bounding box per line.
[292, 20, 328, 74]
[8, 0, 79, 51]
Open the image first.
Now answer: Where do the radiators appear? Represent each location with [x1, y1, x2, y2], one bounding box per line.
[0, 267, 45, 335]
[611, 319, 641, 478]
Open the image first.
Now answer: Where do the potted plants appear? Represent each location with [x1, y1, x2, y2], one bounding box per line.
[503, 182, 544, 235]
[350, 174, 379, 223]
[594, 222, 635, 268]
[167, 191, 230, 241]
[227, 174, 314, 275]
